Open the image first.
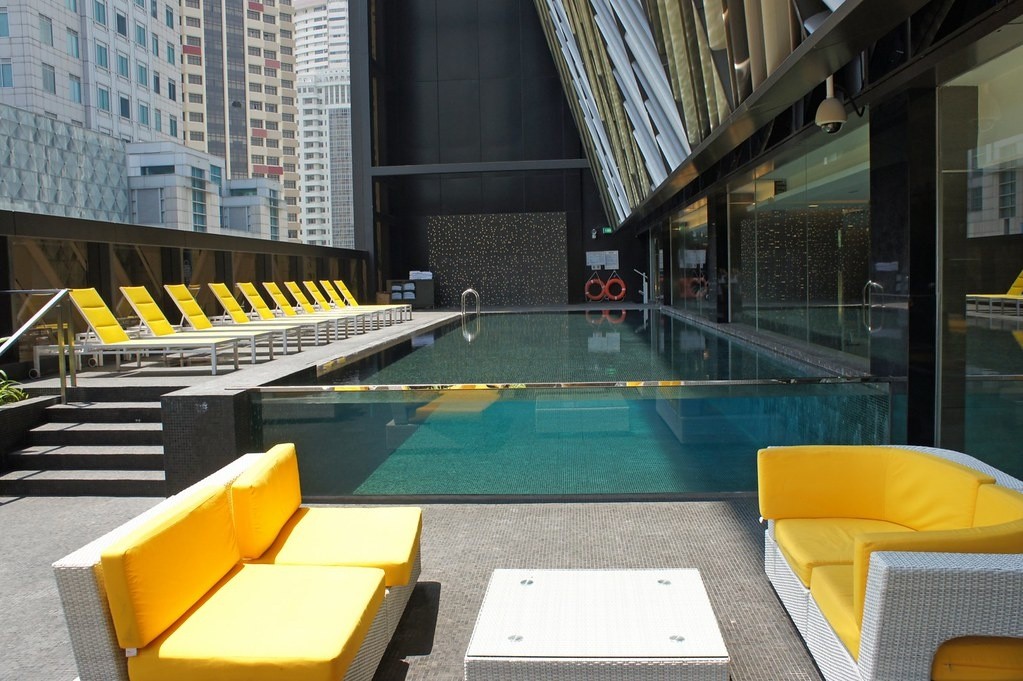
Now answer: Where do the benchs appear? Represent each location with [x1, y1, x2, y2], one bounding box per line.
[756, 445, 1023, 681]
[51, 442, 424, 681]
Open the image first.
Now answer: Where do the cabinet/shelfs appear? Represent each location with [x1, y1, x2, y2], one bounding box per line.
[386, 279, 435, 310]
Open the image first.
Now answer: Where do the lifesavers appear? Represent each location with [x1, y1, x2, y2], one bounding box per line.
[605, 279, 627, 300]
[688, 278, 701, 297]
[606, 309, 627, 323]
[698, 277, 708, 297]
[585, 310, 606, 325]
[655, 278, 663, 300]
[585, 279, 606, 300]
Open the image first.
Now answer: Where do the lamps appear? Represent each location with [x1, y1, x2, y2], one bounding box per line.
[591, 229, 597, 239]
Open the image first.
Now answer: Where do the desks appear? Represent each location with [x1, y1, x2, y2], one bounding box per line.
[465, 567, 732, 681]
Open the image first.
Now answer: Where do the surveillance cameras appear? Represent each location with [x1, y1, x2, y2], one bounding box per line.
[814, 98, 846, 135]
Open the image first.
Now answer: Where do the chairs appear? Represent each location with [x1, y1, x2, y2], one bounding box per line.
[33, 277, 412, 375]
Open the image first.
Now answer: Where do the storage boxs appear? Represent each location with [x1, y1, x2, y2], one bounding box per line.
[376, 290, 391, 305]
